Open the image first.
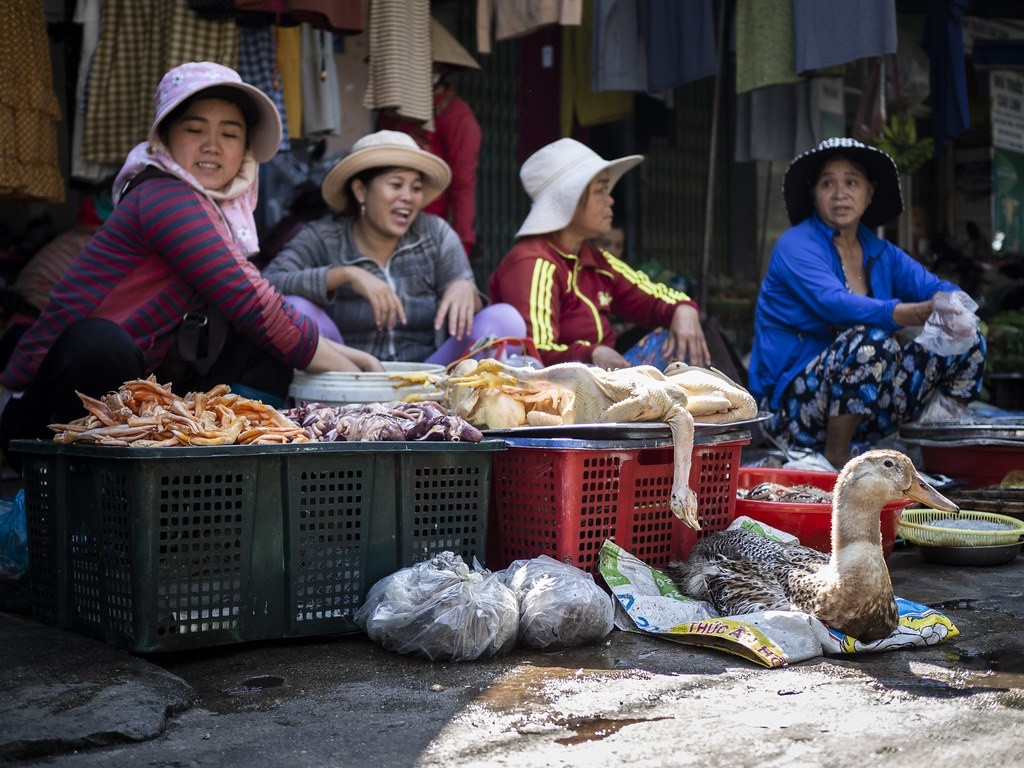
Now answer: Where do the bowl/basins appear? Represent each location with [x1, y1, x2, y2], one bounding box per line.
[730, 469, 913, 572]
[921, 446, 1024, 489]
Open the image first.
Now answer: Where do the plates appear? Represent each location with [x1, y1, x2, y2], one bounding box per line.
[918, 541, 1024, 568]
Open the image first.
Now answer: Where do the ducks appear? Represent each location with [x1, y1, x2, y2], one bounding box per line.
[667, 448, 959, 645]
[388, 352, 757, 536]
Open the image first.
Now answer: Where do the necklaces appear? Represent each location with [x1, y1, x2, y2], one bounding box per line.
[856, 271, 864, 282]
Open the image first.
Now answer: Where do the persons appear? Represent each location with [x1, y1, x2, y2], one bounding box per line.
[749, 136, 988, 473]
[262, 130, 528, 374]
[485, 121, 750, 392]
[0, 59, 382, 567]
[374, 11, 483, 262]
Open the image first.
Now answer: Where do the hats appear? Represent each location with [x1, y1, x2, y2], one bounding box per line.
[147, 61, 282, 163]
[514, 137, 644, 239]
[782, 138, 905, 229]
[432, 16, 481, 69]
[321, 129, 452, 212]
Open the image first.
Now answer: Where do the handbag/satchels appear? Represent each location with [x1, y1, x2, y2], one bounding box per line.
[495, 554, 616, 650]
[355, 552, 519, 662]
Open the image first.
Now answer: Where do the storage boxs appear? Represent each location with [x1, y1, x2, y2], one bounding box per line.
[486, 429, 754, 591]
[8, 439, 507, 661]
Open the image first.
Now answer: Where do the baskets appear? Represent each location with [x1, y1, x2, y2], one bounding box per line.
[896, 508, 1024, 548]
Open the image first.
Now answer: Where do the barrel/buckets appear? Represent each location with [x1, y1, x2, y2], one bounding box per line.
[286, 360, 449, 410]
